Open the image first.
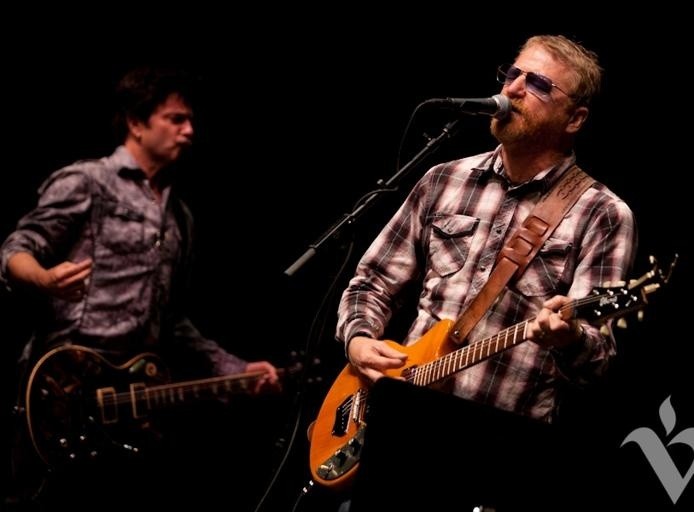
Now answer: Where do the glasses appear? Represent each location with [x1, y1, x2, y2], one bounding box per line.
[496, 62, 574, 104]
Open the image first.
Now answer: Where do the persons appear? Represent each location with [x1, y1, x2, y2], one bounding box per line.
[0, 64, 286, 512]
[334, 34, 639, 512]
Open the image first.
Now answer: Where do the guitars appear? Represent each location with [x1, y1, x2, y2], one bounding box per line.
[310, 253, 679, 490]
[14, 342, 322, 478]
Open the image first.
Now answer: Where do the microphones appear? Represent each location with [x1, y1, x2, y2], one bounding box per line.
[426, 92, 513, 120]
